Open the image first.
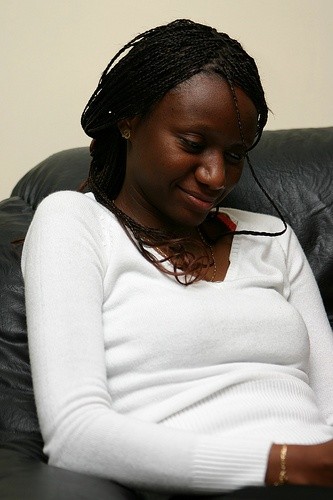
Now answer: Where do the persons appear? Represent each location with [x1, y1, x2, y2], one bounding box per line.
[20, 18, 333, 499]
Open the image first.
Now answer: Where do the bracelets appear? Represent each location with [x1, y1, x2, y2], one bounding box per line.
[274, 443, 293, 488]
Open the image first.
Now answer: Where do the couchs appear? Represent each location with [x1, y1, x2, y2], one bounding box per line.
[0, 125, 333, 500]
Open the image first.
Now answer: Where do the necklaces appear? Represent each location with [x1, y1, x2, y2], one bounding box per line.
[132, 225, 218, 283]
